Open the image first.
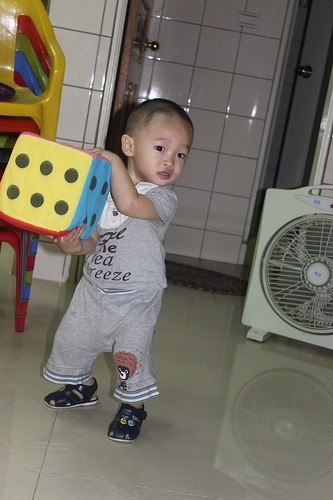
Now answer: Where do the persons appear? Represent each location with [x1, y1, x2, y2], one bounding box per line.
[43, 98, 194, 443]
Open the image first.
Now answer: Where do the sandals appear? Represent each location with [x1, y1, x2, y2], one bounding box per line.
[43, 377, 99, 408]
[107, 403, 147, 442]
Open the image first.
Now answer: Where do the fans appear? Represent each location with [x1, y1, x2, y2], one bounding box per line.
[240, 185, 333, 350]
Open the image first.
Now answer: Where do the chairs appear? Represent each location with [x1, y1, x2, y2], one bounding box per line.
[0, 0, 66, 334]
